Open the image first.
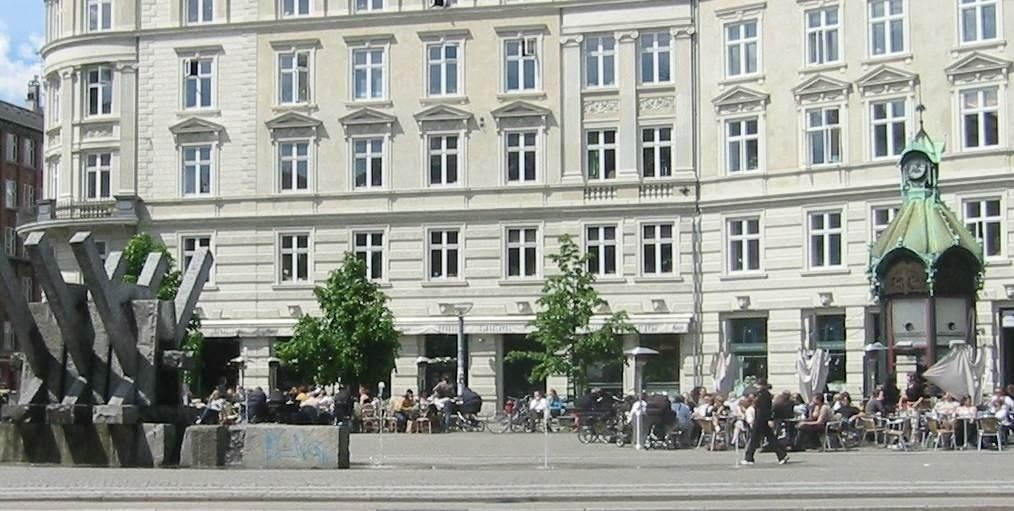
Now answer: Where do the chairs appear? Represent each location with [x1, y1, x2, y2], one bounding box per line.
[573, 391, 1014, 451]
[241, 390, 578, 435]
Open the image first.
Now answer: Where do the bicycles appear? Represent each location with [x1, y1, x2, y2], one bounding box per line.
[486, 394, 632, 447]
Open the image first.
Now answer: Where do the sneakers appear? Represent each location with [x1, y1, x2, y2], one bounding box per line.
[740, 454, 791, 466]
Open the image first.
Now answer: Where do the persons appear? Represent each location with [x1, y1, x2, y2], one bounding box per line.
[737, 379, 792, 467]
[528, 389, 562, 432]
[182, 384, 373, 433]
[393, 389, 452, 432]
[770, 388, 1012, 449]
[573, 386, 755, 448]
